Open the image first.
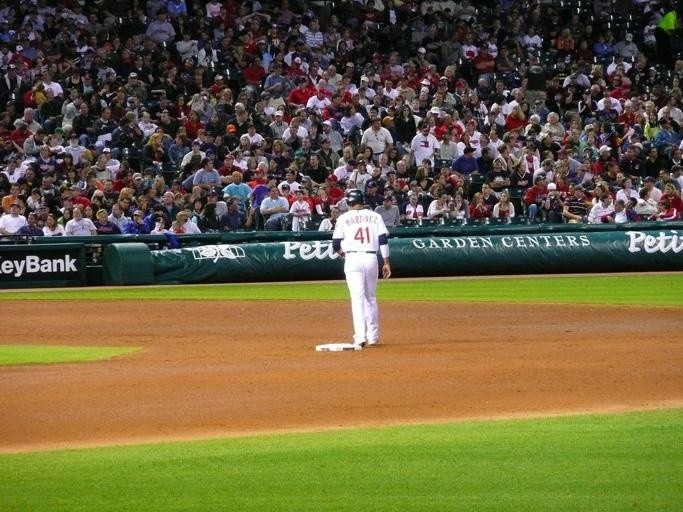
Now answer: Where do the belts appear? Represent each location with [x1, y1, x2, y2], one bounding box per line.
[347, 250, 376, 253]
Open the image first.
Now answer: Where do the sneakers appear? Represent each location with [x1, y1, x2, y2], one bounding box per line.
[355, 338, 367, 346]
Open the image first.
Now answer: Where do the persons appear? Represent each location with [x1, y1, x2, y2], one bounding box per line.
[331, 188, 393, 346]
[0, 0, 683, 241]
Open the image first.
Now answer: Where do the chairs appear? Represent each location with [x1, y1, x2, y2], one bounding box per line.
[515, 0, 674, 87]
[436, 156, 523, 207]
[120, 144, 224, 201]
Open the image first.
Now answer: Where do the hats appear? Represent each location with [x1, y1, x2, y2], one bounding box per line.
[134, 209, 143, 217]
[383, 117, 394, 124]
[255, 167, 263, 174]
[381, 196, 393, 201]
[321, 120, 332, 128]
[430, 106, 440, 115]
[224, 154, 235, 160]
[464, 147, 477, 155]
[346, 61, 353, 68]
[274, 111, 285, 118]
[419, 78, 431, 85]
[368, 181, 378, 187]
[227, 125, 237, 132]
[418, 47, 427, 53]
[294, 56, 301, 63]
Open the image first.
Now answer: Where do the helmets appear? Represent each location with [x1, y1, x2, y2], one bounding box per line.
[346, 190, 364, 205]
